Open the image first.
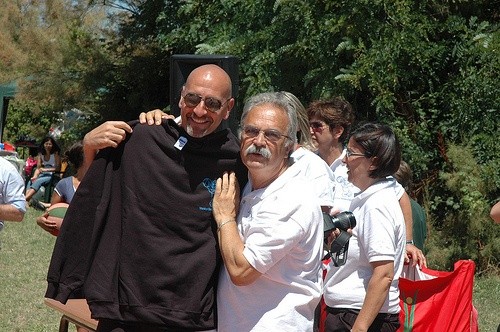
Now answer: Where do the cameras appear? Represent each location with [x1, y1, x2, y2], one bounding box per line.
[323, 211, 356, 244]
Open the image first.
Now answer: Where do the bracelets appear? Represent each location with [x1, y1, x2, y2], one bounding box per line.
[216, 219, 236, 233]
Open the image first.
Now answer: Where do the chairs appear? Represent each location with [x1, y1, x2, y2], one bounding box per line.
[24, 164, 60, 203]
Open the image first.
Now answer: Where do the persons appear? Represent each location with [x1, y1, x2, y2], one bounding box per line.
[0, 64, 500, 332]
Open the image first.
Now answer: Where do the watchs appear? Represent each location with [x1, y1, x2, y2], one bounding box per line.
[406, 240, 416, 245]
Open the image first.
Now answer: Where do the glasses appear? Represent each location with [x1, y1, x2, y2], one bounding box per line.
[238, 124, 293, 143]
[181, 91, 234, 112]
[308, 120, 330, 132]
[345, 147, 372, 158]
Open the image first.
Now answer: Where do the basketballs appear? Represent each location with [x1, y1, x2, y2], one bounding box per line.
[47, 202, 70, 236]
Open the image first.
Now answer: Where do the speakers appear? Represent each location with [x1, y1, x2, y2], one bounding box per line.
[170, 54, 238, 136]
